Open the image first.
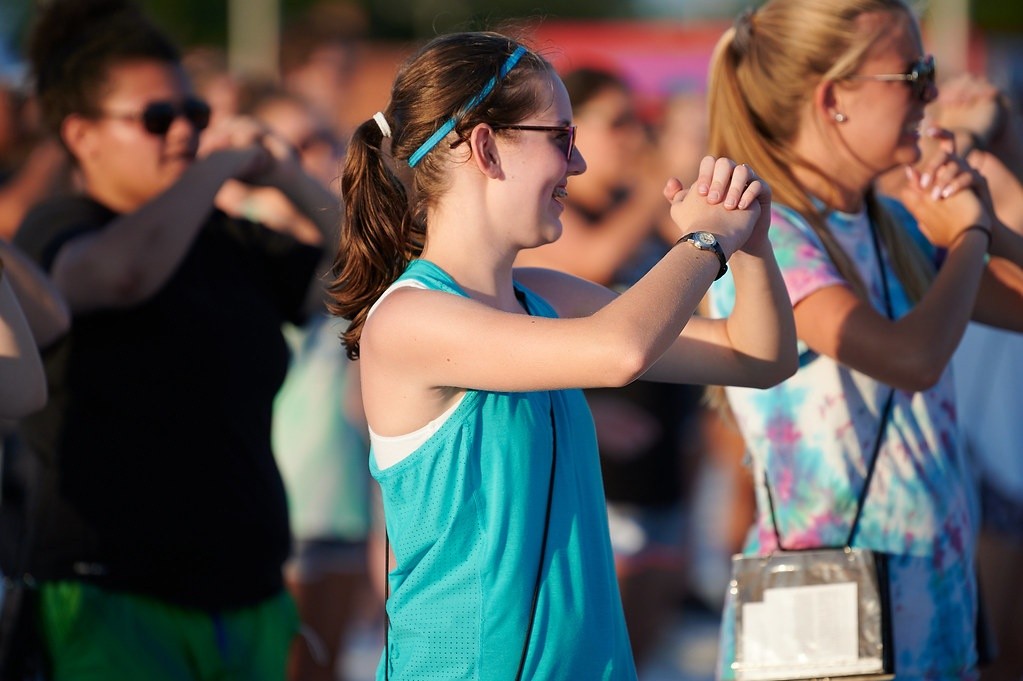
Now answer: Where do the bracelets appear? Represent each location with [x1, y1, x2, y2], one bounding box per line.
[947, 224, 993, 252]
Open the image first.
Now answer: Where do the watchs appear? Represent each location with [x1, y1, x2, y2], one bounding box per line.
[675, 231, 728, 281]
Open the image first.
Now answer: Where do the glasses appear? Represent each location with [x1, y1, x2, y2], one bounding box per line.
[80, 97, 213, 138]
[847, 54, 937, 99]
[448, 119, 577, 162]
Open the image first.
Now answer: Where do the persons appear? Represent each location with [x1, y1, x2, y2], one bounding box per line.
[326, 31, 800, 681]
[0, 54, 705, 681]
[9, 0, 344, 680]
[680, 0, 1023, 681]
